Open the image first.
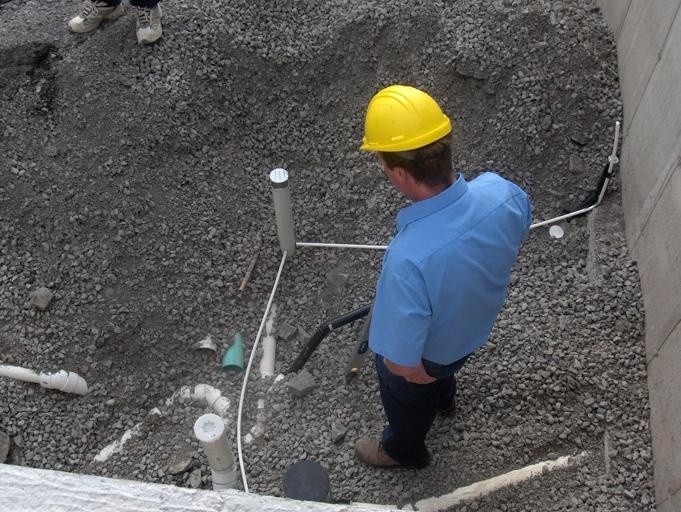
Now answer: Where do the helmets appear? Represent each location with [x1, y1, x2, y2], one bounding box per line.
[361, 85, 454, 154]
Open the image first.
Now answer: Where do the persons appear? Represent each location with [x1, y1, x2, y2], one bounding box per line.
[68, 0, 163, 46]
[356, 85, 531, 468]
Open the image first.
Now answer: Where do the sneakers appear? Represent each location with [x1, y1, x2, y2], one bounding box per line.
[355, 438, 428, 470]
[67, 1, 124, 35]
[136, 5, 162, 45]
